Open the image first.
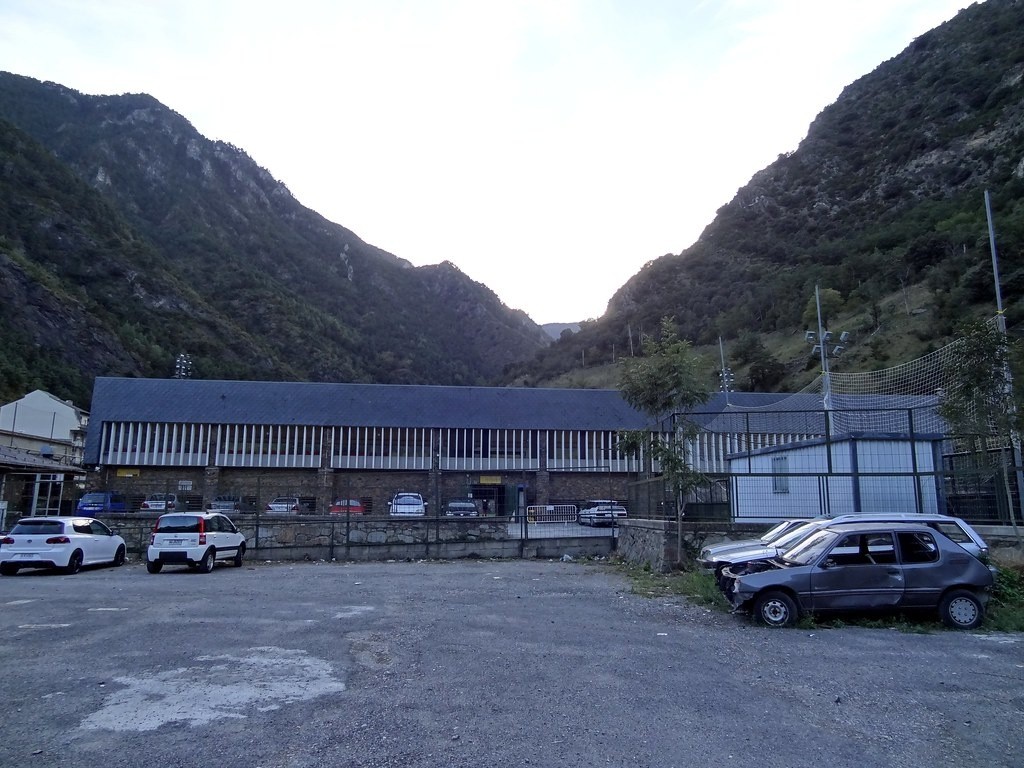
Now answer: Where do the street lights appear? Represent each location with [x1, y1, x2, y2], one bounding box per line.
[804, 284, 850, 437]
[717, 336, 735, 404]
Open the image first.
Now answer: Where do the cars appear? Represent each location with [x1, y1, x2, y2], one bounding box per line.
[441, 497, 480, 517]
[204, 494, 251, 514]
[695, 512, 990, 607]
[75, 491, 135, 519]
[265, 496, 311, 515]
[146, 512, 247, 574]
[577, 499, 628, 527]
[387, 492, 429, 518]
[0, 515, 127, 576]
[731, 521, 996, 629]
[139, 492, 191, 513]
[328, 494, 368, 516]
